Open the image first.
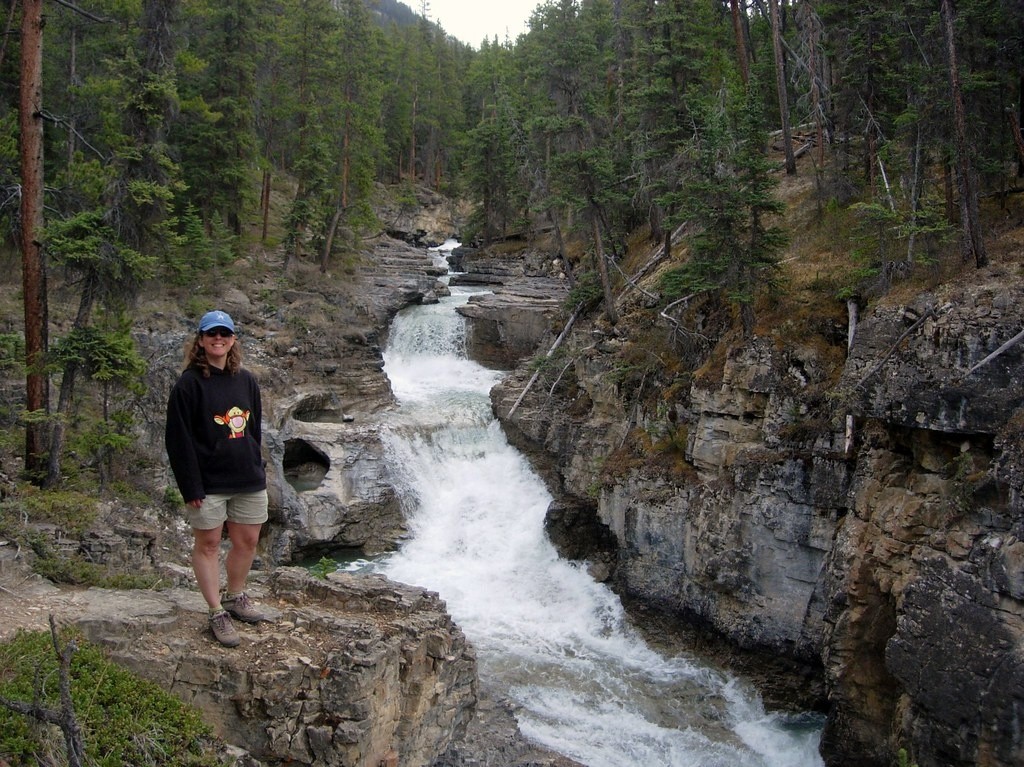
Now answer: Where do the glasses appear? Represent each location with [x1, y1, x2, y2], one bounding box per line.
[203, 329, 232, 337]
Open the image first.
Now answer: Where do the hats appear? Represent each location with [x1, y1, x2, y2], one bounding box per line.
[199, 310, 235, 334]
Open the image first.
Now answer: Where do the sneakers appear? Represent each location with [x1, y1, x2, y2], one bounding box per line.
[208, 610, 241, 647]
[221, 591, 261, 623]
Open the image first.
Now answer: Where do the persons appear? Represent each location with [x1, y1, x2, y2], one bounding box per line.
[165, 311, 268, 647]
